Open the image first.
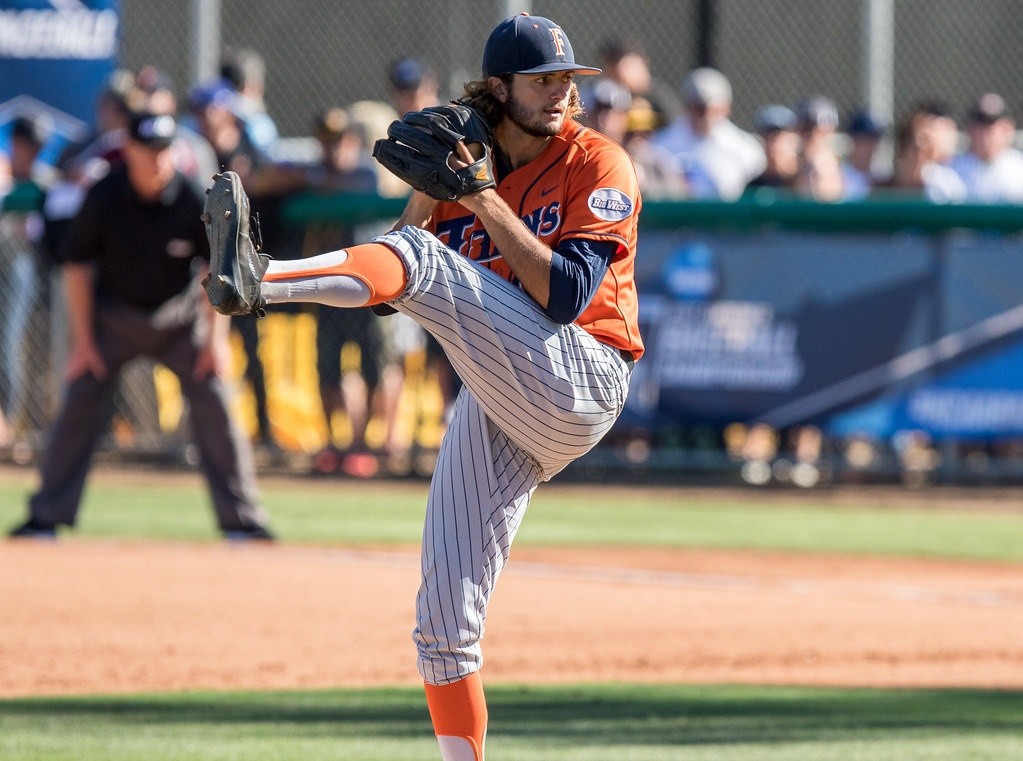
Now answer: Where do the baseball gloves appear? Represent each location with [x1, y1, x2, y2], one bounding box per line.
[370, 97, 497, 205]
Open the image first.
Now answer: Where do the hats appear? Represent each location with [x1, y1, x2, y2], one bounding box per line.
[481, 12, 603, 82]
[684, 69, 734, 105]
[190, 83, 236, 110]
[759, 104, 795, 131]
[388, 57, 425, 86]
[128, 113, 174, 147]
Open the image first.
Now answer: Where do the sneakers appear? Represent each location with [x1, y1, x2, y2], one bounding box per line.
[202, 170, 268, 317]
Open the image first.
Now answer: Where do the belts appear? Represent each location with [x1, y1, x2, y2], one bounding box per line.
[618, 349, 636, 373]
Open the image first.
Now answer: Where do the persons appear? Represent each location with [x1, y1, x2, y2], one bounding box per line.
[11, 32, 1023, 214]
[201, 9, 644, 761]
[5, 111, 281, 545]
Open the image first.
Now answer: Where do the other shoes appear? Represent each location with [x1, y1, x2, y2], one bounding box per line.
[10, 517, 56, 535]
[220, 524, 277, 542]
